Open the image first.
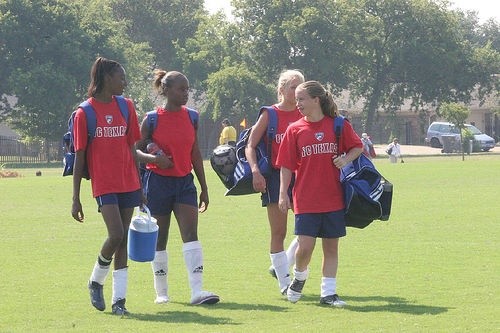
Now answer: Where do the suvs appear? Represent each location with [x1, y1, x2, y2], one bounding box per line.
[425, 121, 496, 151]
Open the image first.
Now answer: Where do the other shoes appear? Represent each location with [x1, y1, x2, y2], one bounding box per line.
[155, 294, 169, 304]
[268, 265, 292, 295]
[191, 291, 219, 304]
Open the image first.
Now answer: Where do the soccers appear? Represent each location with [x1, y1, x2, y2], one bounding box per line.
[210, 144, 236, 174]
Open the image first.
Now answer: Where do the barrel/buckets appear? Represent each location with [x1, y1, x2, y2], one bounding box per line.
[126, 201, 160, 261]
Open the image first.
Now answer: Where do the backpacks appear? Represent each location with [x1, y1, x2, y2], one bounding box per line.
[62, 94, 130, 179]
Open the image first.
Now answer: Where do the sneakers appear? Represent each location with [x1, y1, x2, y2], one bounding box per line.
[112, 297, 131, 316]
[88, 279, 106, 311]
[320, 294, 346, 307]
[287, 278, 306, 303]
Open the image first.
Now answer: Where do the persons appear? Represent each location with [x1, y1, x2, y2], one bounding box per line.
[136, 68, 220, 304]
[219, 119, 236, 145]
[361, 133, 372, 160]
[70, 55, 149, 319]
[244, 69, 306, 301]
[277, 80, 364, 309]
[389, 138, 401, 164]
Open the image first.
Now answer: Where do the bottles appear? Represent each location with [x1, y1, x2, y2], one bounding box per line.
[63, 131, 73, 154]
[145, 139, 166, 157]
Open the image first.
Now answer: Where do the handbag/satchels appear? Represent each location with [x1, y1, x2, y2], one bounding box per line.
[345, 153, 393, 229]
[224, 128, 273, 196]
[385, 143, 392, 154]
